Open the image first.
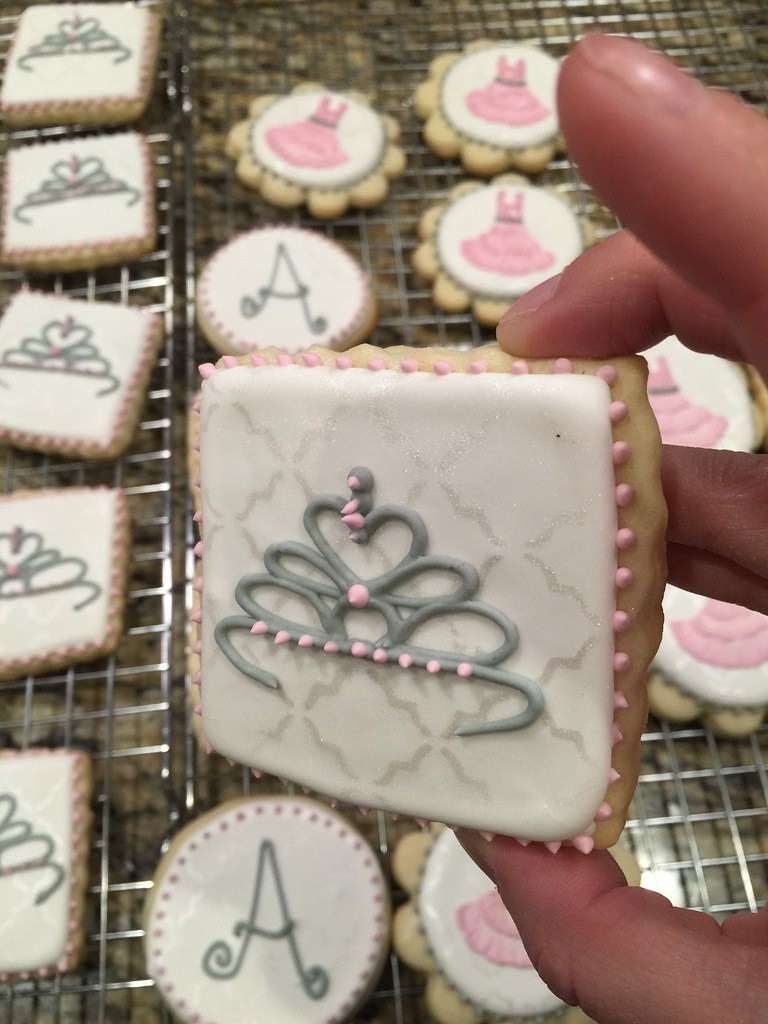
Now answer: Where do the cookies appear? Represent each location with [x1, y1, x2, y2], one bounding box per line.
[0, 0, 768, 1024]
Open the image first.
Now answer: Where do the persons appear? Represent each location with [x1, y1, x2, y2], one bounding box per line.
[452, 33, 768, 1024]
